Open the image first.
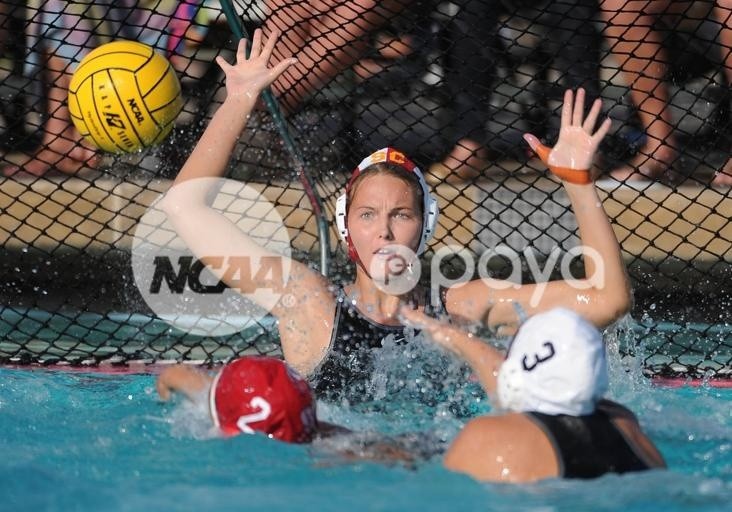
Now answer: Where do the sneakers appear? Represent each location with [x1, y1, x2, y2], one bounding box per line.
[424, 157, 487, 184]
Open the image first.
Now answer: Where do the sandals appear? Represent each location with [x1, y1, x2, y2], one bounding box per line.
[596, 158, 689, 188]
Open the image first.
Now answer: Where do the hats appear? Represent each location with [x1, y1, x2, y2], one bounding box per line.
[336, 145, 440, 259]
[209, 354, 319, 444]
[495, 308, 609, 417]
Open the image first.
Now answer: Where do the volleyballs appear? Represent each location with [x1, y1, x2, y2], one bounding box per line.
[68, 41, 181, 155]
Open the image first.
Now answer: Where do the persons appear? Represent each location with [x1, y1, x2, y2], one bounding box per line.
[160, 21, 643, 414]
[384, 296, 670, 486]
[156, 358, 421, 485]
